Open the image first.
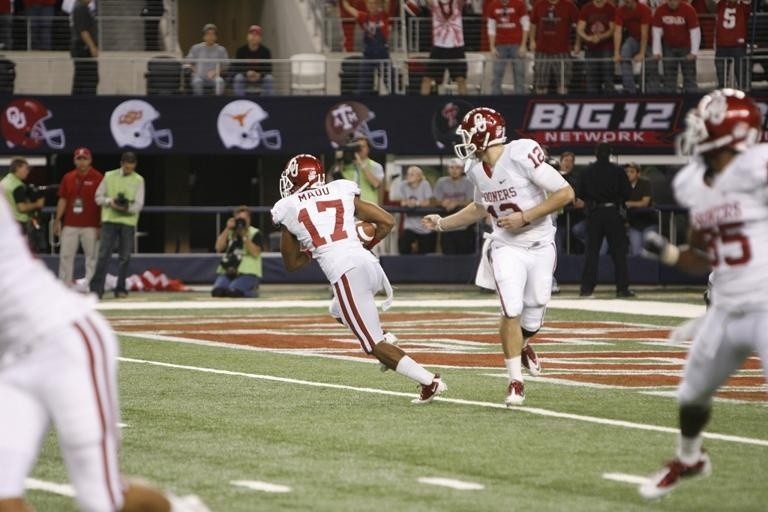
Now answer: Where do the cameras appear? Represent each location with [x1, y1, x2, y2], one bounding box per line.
[229, 217, 246, 232]
[113, 192, 129, 208]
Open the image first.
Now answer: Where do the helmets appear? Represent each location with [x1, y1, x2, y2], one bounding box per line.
[686, 88, 761, 154]
[280, 154, 325, 198]
[454, 107, 507, 160]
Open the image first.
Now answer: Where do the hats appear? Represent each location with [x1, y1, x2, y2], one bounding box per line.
[249, 25, 263, 34]
[73, 148, 90, 160]
[203, 23, 216, 32]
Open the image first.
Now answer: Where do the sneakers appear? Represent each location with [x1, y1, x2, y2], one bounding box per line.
[639, 448, 711, 499]
[521, 345, 541, 376]
[505, 380, 525, 405]
[411, 373, 446, 403]
[380, 333, 397, 372]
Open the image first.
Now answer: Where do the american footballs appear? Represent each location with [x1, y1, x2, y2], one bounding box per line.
[355, 221, 375, 244]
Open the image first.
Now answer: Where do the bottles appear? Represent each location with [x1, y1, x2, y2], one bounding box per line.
[430, 75, 438, 94]
[446, 77, 453, 96]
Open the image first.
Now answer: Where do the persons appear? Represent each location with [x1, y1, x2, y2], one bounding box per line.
[3, 0, 749, 97]
[331, 138, 480, 256]
[418, 108, 579, 407]
[268, 150, 452, 407]
[634, 84, 767, 498]
[3, 147, 146, 299]
[0, 195, 211, 511]
[211, 206, 265, 298]
[539, 145, 710, 298]
